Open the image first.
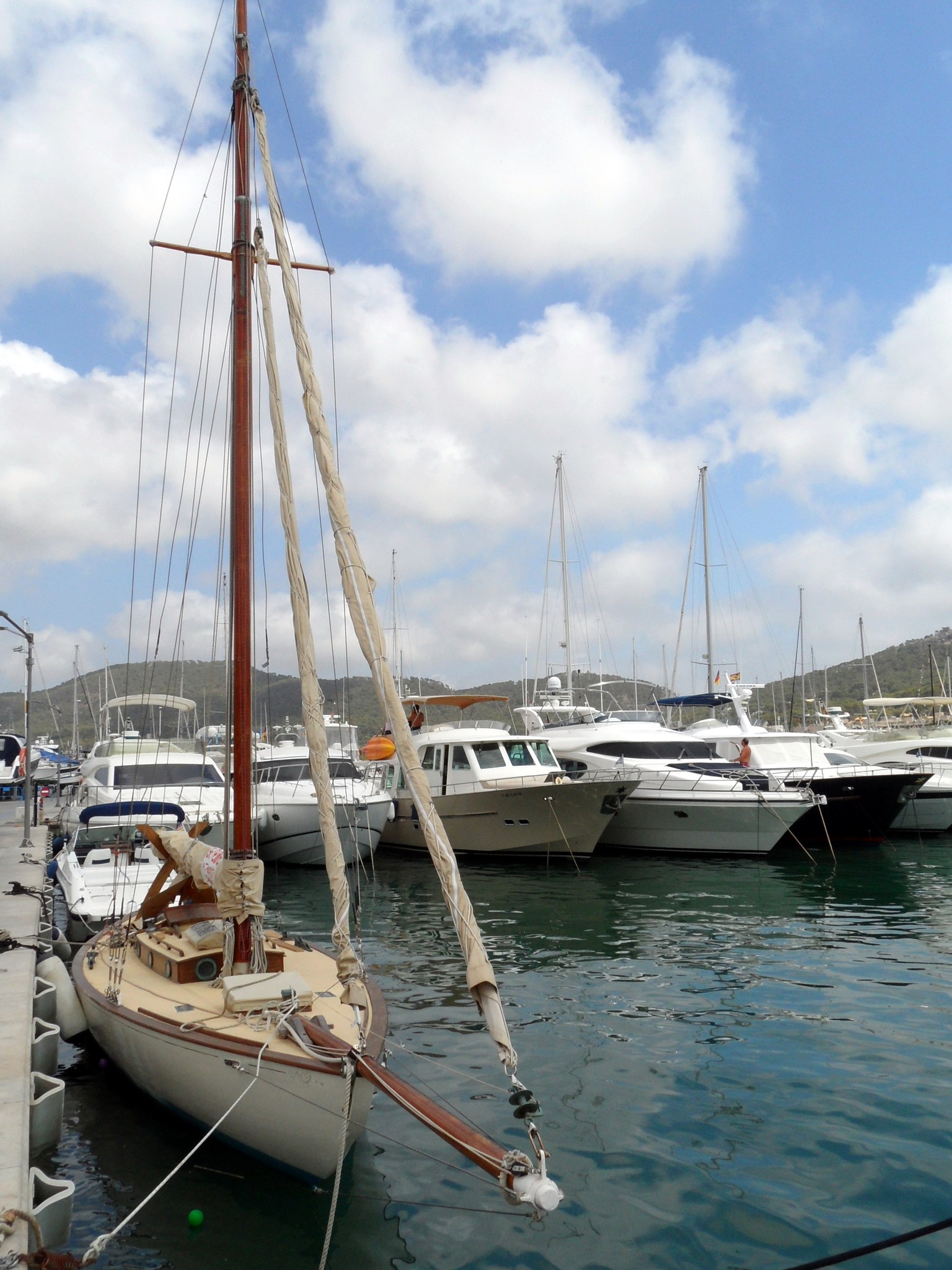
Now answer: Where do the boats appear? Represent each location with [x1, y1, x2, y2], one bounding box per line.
[54, 801, 189, 943]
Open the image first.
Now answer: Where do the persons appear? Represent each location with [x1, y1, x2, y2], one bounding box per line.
[729, 738, 751, 768]
[407, 704, 424, 731]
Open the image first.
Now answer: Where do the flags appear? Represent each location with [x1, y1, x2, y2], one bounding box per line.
[730, 672, 741, 681]
[715, 670, 720, 687]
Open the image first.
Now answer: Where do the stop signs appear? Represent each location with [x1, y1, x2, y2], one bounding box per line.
[41, 787, 50, 797]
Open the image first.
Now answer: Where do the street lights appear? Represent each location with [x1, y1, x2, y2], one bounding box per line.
[158, 706, 163, 736]
[76, 700, 82, 759]
[0, 609, 36, 848]
[30, 700, 62, 808]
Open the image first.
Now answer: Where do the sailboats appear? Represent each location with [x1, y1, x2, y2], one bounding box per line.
[71, 0, 565, 1232]
[0, 447, 952, 871]
